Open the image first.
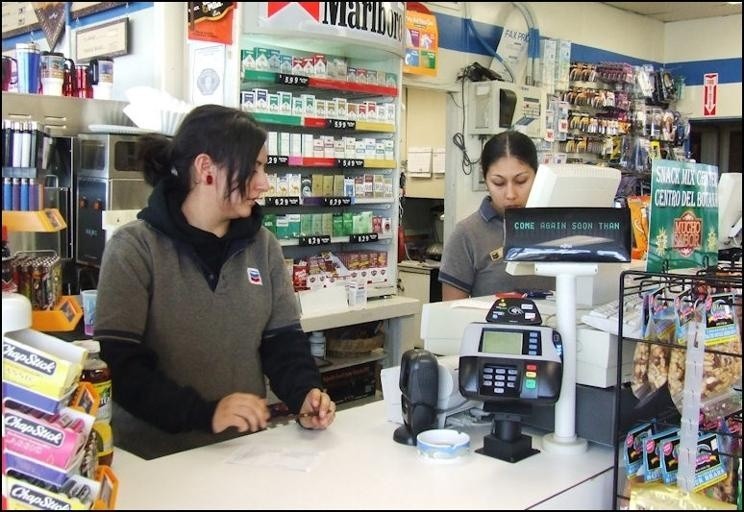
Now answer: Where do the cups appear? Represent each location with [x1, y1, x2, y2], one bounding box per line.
[81, 288, 102, 337]
[2, 40, 113, 98]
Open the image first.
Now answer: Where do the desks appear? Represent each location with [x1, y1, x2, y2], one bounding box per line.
[99, 397, 618, 510]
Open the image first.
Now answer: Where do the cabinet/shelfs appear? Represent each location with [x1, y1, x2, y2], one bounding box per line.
[614, 265, 744, 508]
[191, 2, 404, 295]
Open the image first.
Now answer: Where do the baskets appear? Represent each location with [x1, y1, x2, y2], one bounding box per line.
[326, 328, 385, 359]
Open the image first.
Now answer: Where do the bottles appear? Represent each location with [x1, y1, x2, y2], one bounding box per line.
[309, 331, 328, 360]
[80, 340, 113, 468]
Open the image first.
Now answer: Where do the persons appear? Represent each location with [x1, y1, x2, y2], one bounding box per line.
[91, 107, 336, 461]
[438, 132, 556, 301]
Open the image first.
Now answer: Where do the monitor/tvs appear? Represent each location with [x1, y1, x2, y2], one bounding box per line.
[506, 164, 621, 276]
